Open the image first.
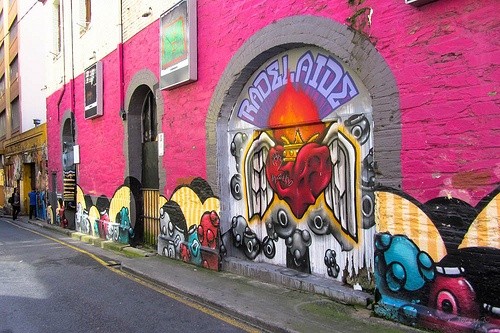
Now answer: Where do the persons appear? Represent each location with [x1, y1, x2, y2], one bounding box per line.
[12, 187, 21, 220]
[28, 188, 38, 221]
